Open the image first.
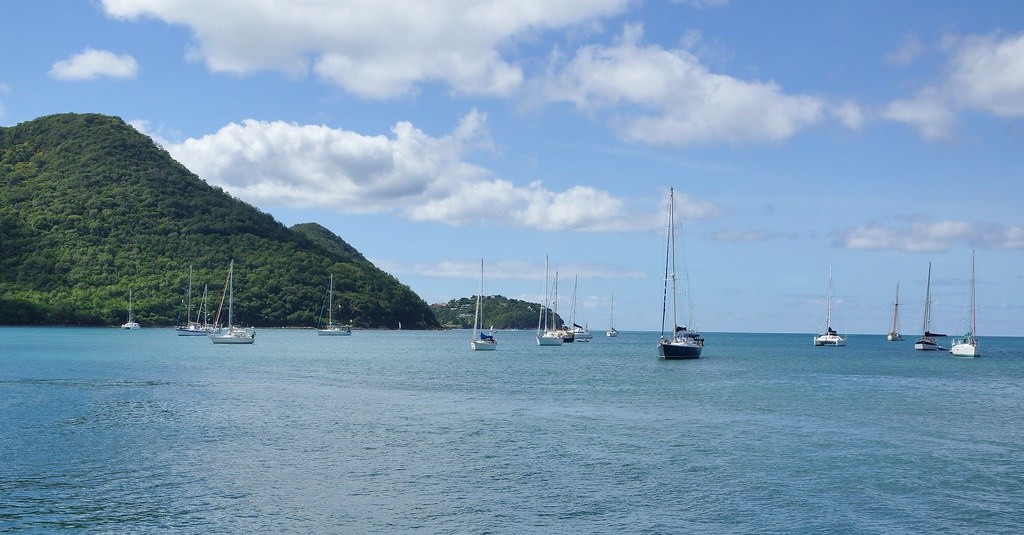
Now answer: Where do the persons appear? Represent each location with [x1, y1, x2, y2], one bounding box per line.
[251, 326, 256, 338]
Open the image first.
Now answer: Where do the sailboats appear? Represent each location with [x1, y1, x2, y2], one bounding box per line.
[657, 186, 705, 359]
[888, 280, 902, 341]
[535, 254, 594, 346]
[174, 259, 257, 345]
[471, 257, 498, 351]
[315, 272, 353, 337]
[948, 248, 979, 357]
[914, 260, 939, 350]
[813, 264, 849, 347]
[120, 288, 141, 331]
[606, 291, 620, 337]
